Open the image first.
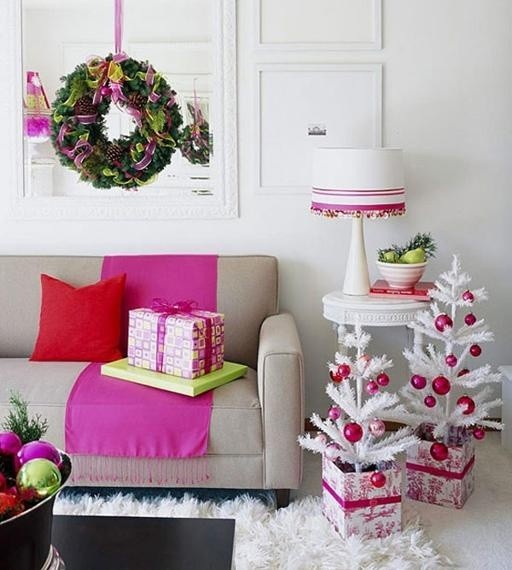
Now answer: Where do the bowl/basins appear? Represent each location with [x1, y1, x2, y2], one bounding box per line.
[374, 256, 428, 292]
[0, 449, 73, 570]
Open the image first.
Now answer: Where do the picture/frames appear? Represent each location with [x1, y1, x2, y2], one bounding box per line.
[253, 64, 385, 202]
[249, 0, 384, 53]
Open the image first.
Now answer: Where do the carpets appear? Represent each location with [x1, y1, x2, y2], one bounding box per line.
[56, 490, 455, 570]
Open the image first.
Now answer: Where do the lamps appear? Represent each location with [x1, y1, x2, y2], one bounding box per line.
[303, 146, 408, 298]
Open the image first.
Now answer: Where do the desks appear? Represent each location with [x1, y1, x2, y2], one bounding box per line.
[318, 289, 439, 360]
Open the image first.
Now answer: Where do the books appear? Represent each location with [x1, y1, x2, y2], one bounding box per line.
[368, 280, 434, 301]
[100, 359, 250, 397]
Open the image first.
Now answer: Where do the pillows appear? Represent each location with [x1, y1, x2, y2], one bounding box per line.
[28, 271, 126, 365]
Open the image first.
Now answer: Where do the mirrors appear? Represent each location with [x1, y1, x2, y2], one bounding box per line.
[11, 1, 240, 221]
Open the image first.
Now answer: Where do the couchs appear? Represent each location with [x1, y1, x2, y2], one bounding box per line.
[0, 252, 308, 510]
